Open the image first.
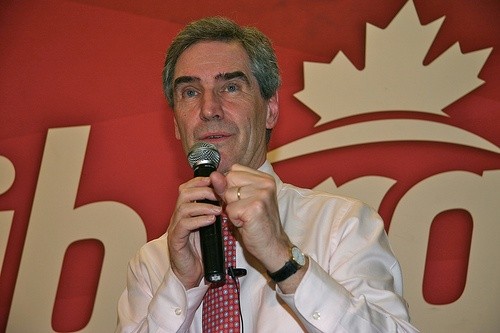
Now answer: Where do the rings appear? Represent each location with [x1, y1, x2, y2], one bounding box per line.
[237, 186, 240, 200]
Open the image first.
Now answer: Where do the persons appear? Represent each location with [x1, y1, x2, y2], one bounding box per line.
[112, 18, 422, 333]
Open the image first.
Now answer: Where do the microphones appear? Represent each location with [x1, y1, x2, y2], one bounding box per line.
[228, 266, 236, 279]
[186, 142, 226, 283]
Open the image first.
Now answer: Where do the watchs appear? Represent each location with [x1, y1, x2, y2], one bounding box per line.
[266, 245, 305, 282]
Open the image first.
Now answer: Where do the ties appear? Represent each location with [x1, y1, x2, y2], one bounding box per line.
[202, 202, 240, 333]
